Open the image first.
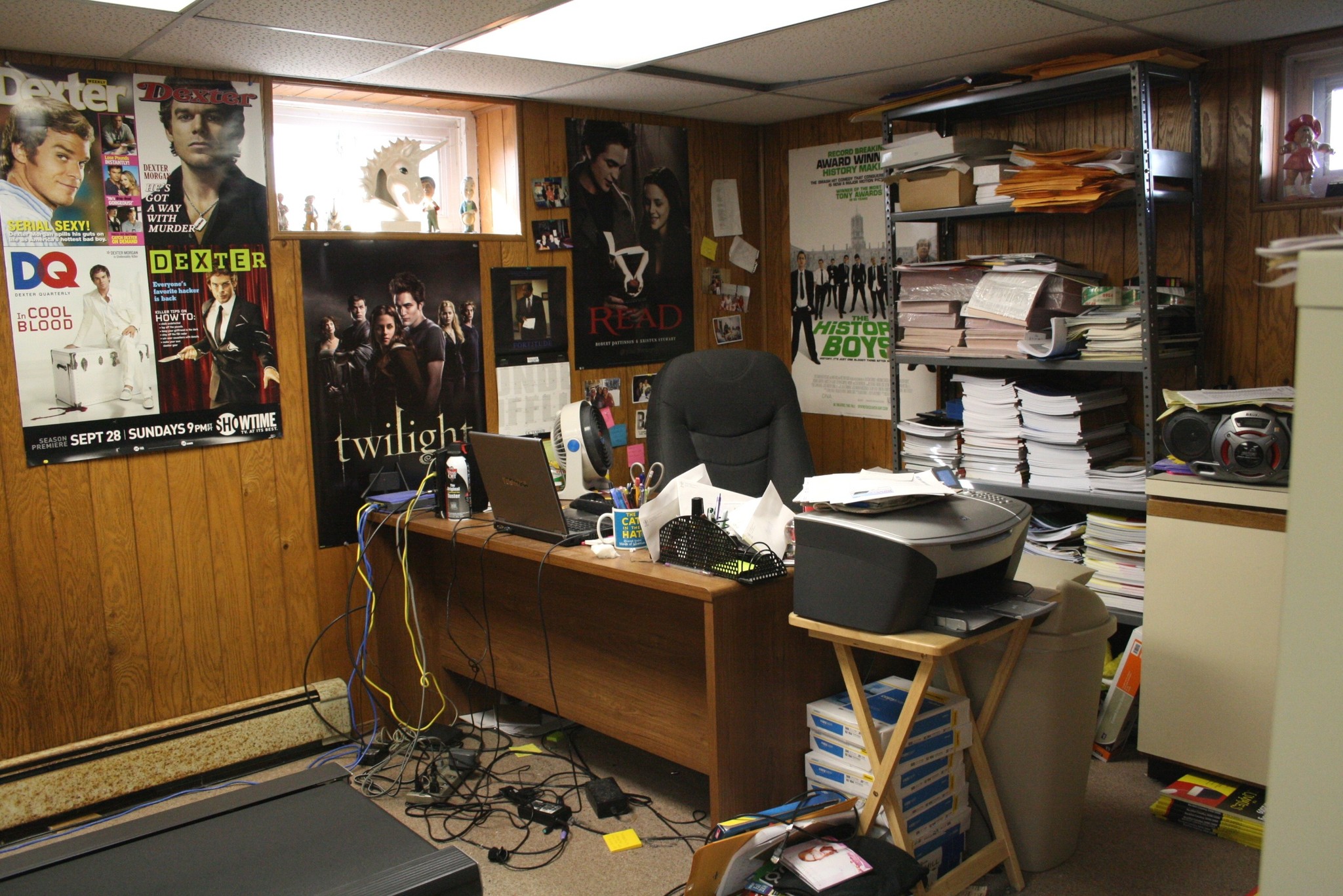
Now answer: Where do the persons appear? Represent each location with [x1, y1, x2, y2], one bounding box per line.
[313, 272, 481, 421]
[537, 234, 567, 250]
[141, 76, 270, 244]
[101, 115, 135, 153]
[515, 282, 548, 341]
[571, 119, 691, 326]
[460, 176, 477, 233]
[63, 264, 154, 411]
[1279, 115, 1335, 200]
[107, 206, 143, 233]
[542, 183, 567, 208]
[789, 238, 939, 365]
[420, 176, 441, 233]
[304, 195, 318, 230]
[277, 193, 289, 231]
[635, 379, 651, 401]
[0, 96, 96, 245]
[176, 261, 280, 409]
[710, 274, 746, 342]
[105, 166, 140, 196]
[588, 384, 615, 409]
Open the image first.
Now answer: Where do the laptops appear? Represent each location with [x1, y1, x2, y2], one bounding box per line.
[468, 431, 613, 547]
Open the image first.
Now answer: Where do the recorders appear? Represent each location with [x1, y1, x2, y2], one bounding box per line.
[1162, 404, 1294, 488]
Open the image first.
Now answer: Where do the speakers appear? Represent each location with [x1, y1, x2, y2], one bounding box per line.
[434, 442, 489, 517]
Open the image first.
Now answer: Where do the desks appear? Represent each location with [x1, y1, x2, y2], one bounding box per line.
[788, 585, 1060, 896]
[365, 507, 869, 847]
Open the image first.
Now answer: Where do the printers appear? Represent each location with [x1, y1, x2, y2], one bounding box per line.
[793, 465, 1058, 640]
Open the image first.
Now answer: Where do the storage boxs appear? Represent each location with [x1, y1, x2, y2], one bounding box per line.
[1092, 625, 1143, 762]
[879, 136, 1020, 213]
[804, 675, 972, 889]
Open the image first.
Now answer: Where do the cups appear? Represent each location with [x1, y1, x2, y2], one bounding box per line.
[596, 506, 646, 550]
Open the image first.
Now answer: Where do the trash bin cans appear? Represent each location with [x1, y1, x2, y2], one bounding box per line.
[929, 555, 1118, 873]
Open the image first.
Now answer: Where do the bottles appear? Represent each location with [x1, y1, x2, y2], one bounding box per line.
[444, 443, 472, 521]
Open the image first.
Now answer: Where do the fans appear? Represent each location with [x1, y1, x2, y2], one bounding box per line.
[550, 398, 615, 500]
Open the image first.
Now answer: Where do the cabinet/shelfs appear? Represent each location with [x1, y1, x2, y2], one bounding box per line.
[882, 60, 1206, 628]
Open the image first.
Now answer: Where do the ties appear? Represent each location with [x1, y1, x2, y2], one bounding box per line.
[528, 298, 530, 313]
[800, 272, 806, 299]
[821, 269, 824, 286]
[873, 266, 876, 280]
[845, 265, 848, 273]
[214, 305, 224, 347]
[857, 264, 860, 269]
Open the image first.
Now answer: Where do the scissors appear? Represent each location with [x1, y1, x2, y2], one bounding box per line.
[630, 461, 665, 496]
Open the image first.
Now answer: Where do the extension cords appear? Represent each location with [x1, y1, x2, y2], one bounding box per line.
[405, 752, 479, 805]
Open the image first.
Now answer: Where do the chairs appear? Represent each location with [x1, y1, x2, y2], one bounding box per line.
[646, 348, 819, 512]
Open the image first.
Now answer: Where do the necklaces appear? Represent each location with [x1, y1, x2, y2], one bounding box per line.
[183, 187, 220, 232]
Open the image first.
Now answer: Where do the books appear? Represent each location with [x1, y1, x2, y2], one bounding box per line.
[898, 253, 1206, 362]
[1148, 771, 1267, 850]
[1023, 508, 1148, 614]
[897, 373, 1147, 498]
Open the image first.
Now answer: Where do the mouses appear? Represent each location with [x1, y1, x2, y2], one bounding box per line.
[579, 492, 604, 500]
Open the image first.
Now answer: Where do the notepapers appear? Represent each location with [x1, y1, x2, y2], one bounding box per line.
[602, 827, 643, 853]
[713, 559, 758, 575]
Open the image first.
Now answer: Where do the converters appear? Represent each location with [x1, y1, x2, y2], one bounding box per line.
[584, 777, 630, 819]
[410, 721, 463, 747]
[517, 797, 572, 829]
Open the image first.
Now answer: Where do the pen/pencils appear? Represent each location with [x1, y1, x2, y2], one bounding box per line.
[664, 561, 712, 575]
[722, 510, 728, 532]
[581, 535, 614, 545]
[714, 492, 721, 519]
[710, 509, 716, 523]
[608, 470, 653, 509]
[702, 514, 707, 520]
[708, 507, 713, 521]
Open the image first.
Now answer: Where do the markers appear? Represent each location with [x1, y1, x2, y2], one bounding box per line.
[692, 497, 704, 517]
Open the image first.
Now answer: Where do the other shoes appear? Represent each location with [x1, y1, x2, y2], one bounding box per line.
[120, 390, 132, 400]
[144, 397, 154, 408]
[811, 358, 820, 364]
[813, 302, 888, 320]
[792, 357, 795, 364]
